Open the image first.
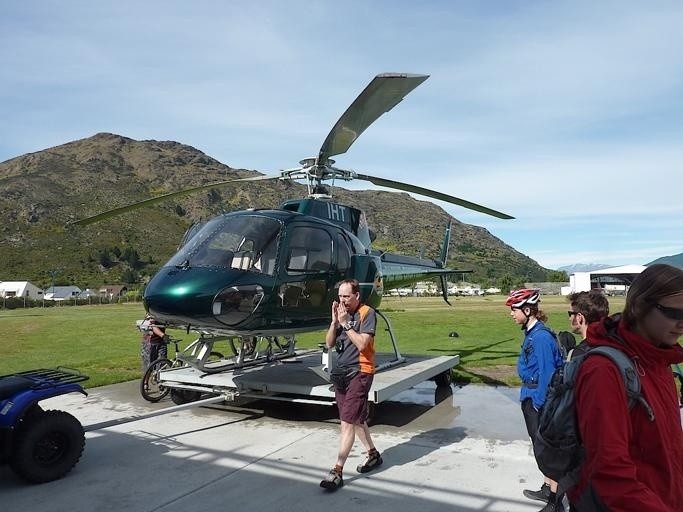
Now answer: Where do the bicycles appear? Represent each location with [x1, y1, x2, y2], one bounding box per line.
[228, 335, 296, 358]
[139, 330, 225, 408]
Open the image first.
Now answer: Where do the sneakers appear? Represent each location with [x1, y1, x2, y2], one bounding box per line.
[523, 482, 553, 503]
[320, 468, 344, 489]
[538, 490, 566, 512]
[356, 451, 384, 474]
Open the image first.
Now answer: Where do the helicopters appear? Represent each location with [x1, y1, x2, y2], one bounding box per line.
[64, 72, 515, 384]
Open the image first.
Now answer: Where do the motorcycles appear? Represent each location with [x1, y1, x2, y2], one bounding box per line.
[0, 364, 92, 487]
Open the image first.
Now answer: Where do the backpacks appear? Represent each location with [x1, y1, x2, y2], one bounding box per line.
[532, 345, 642, 483]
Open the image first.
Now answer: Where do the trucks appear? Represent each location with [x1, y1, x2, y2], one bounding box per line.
[605, 284, 630, 297]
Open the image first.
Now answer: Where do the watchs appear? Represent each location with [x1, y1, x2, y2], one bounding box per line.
[343, 324, 352, 331]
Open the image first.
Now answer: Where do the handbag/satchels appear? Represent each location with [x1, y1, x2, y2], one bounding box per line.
[330, 364, 362, 391]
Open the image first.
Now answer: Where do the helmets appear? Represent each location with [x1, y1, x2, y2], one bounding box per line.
[504, 287, 541, 308]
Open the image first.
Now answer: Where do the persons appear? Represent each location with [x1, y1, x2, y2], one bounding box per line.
[565, 263, 683, 510]
[319, 278, 383, 491]
[141, 316, 170, 392]
[506, 288, 561, 512]
[567, 293, 609, 338]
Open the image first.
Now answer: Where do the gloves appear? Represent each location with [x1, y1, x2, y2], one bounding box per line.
[161, 334, 170, 345]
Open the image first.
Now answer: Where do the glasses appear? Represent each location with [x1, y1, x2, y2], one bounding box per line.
[655, 302, 683, 321]
[568, 311, 579, 317]
